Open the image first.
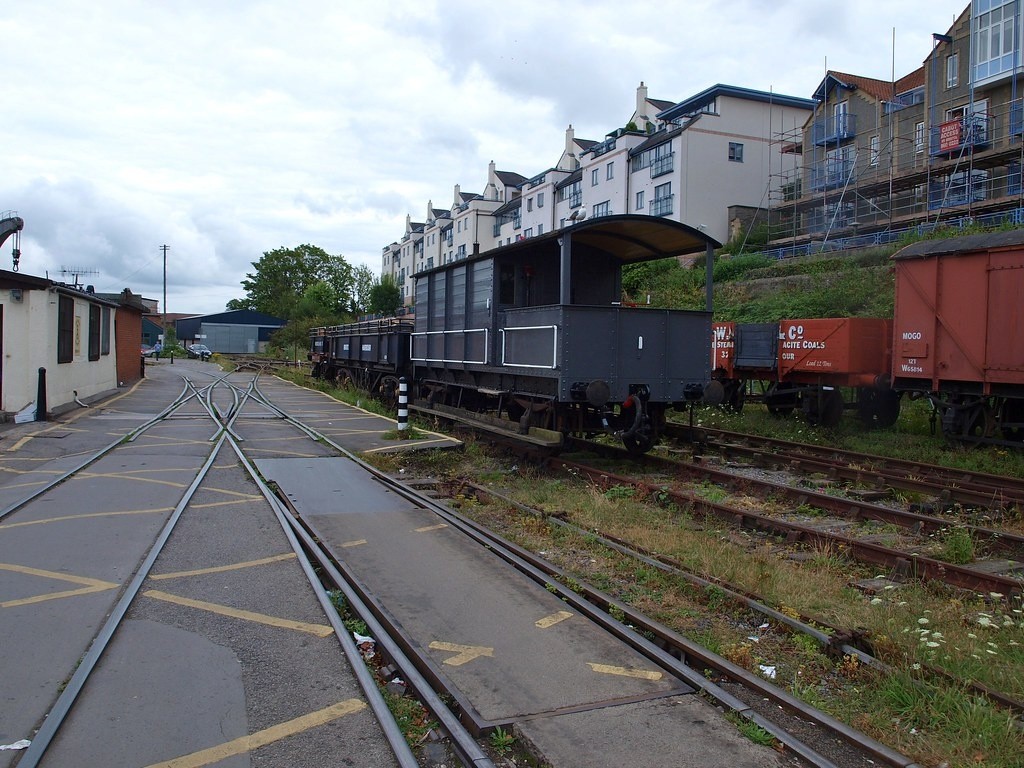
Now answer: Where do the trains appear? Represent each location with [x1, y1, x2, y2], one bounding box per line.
[307, 213, 723, 452]
[713, 224, 1024, 434]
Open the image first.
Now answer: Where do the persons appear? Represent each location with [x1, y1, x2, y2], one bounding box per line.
[153, 340, 161, 361]
[953, 111, 964, 136]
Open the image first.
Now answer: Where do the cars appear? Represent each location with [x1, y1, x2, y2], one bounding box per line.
[143, 346, 160, 358]
[140, 344, 152, 355]
[188, 343, 212, 359]
